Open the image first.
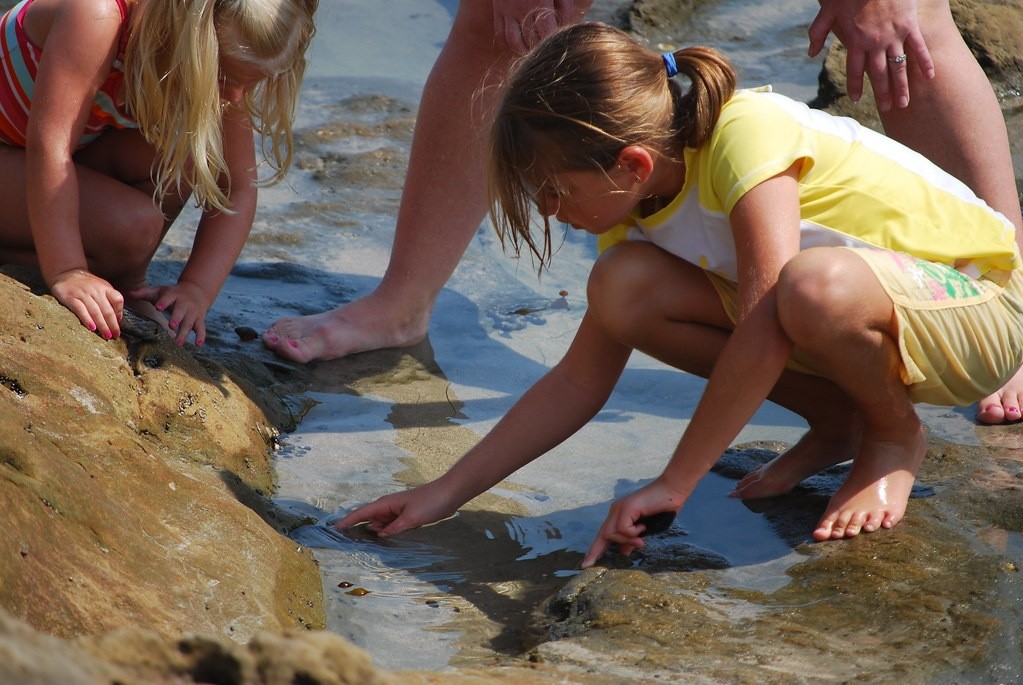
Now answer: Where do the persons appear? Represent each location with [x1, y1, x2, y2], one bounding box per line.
[0, 0, 321, 348]
[260, 0, 1023, 423]
[334, 21, 1023, 570]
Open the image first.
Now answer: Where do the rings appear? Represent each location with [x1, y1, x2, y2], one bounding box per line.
[888, 54, 908, 65]
